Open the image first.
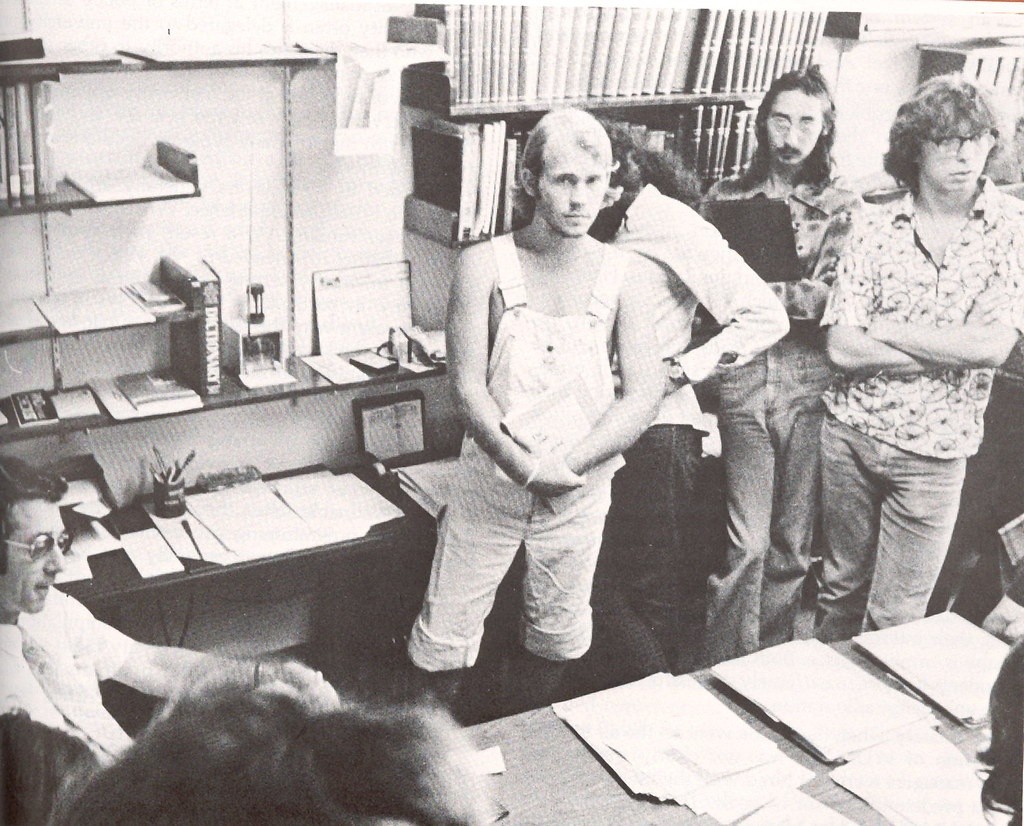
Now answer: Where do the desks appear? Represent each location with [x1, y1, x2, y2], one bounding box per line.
[51, 463, 413, 706]
[459, 641, 1012, 826]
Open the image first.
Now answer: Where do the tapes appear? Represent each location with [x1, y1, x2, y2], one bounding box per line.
[372, 462, 386, 477]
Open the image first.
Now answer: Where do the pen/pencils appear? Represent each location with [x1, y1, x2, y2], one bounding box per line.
[147, 447, 196, 483]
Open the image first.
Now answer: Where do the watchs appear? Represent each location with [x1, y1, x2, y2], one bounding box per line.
[668, 358, 691, 390]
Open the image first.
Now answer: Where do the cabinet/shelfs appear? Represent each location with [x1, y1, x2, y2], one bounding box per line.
[0, 47, 447, 447]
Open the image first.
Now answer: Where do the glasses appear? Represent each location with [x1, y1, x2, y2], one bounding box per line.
[4, 530, 76, 560]
[923, 128, 983, 157]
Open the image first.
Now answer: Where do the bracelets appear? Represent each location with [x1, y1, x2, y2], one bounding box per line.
[253, 657, 261, 690]
[519, 461, 541, 492]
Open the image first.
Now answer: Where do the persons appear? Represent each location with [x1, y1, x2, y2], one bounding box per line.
[810, 69, 1024, 646]
[0, 451, 499, 826]
[694, 66, 865, 672]
[401, 105, 792, 725]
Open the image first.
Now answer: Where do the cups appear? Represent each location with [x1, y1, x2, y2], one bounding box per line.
[153, 470, 186, 518]
